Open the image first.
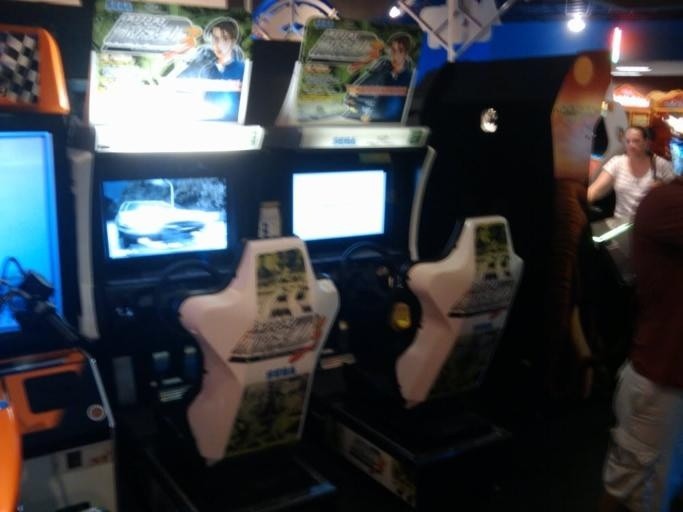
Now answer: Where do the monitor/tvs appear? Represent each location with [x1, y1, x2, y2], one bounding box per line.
[0, 113, 82, 352]
[92, 152, 243, 293]
[277, 151, 400, 253]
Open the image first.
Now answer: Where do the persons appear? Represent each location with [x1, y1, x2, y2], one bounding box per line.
[598, 172, 683, 512]
[586, 124, 676, 276]
[343, 31, 414, 123]
[174, 16, 245, 123]
